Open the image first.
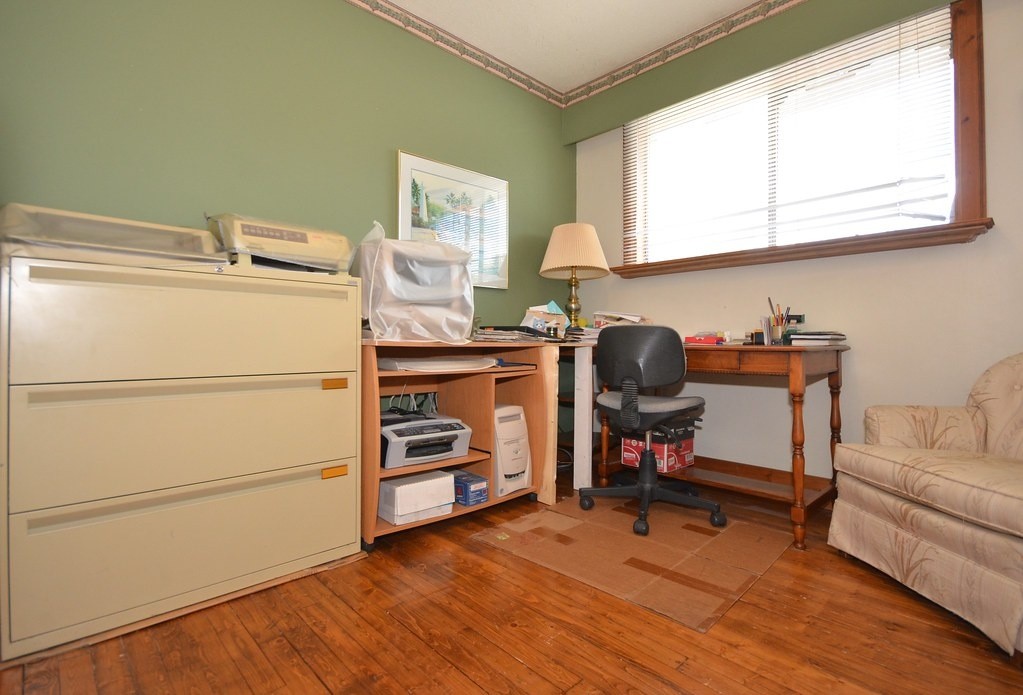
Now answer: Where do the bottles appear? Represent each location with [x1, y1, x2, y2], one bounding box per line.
[783, 320, 798, 345]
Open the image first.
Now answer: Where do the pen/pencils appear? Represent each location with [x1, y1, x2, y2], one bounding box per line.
[766, 296, 790, 326]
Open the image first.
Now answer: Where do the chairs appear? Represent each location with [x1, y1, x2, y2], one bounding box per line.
[579, 326, 727, 536]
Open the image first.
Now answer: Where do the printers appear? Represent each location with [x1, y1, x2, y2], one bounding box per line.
[380, 409, 473, 470]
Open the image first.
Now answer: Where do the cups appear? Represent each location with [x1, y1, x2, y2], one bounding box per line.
[770, 325, 784, 346]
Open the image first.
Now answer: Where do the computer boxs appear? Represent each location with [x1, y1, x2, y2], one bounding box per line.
[494, 403, 533, 498]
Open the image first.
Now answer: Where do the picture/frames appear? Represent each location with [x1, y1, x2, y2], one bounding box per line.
[396, 148, 509, 290]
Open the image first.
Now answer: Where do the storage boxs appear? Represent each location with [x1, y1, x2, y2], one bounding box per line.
[621, 427, 695, 473]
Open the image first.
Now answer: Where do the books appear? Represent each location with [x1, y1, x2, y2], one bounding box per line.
[476, 325, 602, 343]
[790, 332, 846, 346]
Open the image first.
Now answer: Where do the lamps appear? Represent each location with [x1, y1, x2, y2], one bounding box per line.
[539, 223, 611, 327]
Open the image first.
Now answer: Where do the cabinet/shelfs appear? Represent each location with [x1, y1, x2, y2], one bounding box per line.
[362, 339, 559, 552]
[559, 343, 618, 450]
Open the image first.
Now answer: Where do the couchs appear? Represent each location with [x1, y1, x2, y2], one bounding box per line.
[827, 352, 1023, 657]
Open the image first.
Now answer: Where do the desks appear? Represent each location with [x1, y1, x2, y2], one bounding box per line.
[599, 343, 851, 549]
